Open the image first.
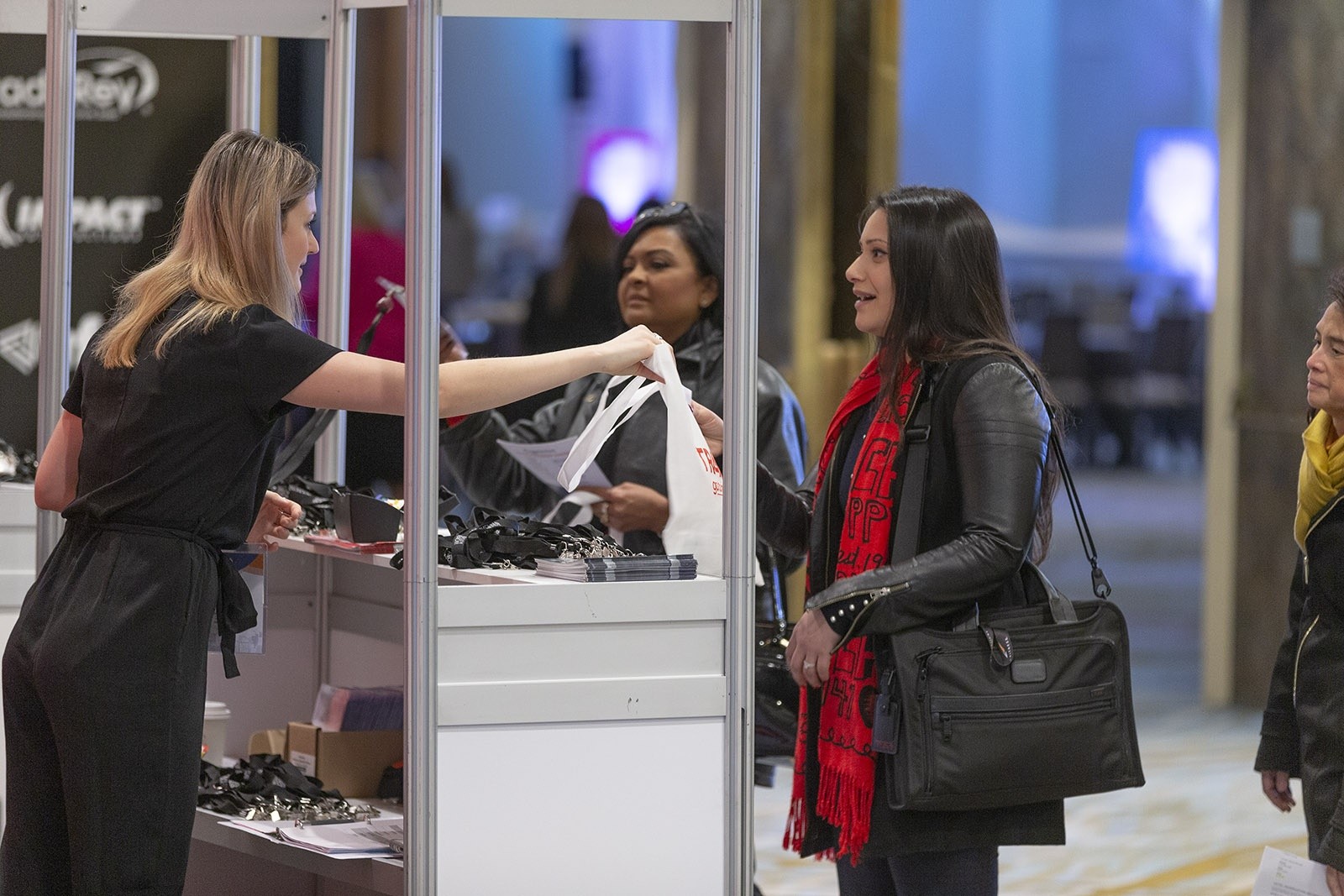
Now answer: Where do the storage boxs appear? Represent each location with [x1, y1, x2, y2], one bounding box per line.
[288, 719, 404, 798]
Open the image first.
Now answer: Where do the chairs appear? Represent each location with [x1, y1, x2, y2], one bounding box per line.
[1013, 277, 1200, 478]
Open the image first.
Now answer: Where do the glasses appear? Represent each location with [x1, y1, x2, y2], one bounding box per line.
[628, 202, 715, 255]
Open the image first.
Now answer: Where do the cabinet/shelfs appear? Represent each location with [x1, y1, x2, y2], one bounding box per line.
[182, 521, 728, 895]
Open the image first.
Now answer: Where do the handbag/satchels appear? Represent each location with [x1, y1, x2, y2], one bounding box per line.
[643, 343, 765, 588]
[888, 351, 1145, 809]
[754, 624, 805, 757]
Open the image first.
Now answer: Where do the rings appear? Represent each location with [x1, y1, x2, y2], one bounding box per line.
[803, 663, 815, 668]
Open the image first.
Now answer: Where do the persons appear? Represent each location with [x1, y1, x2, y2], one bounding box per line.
[1253, 270, 1344, 896]
[0, 129, 667, 896]
[690, 184, 1066, 896]
[438, 201, 806, 896]
